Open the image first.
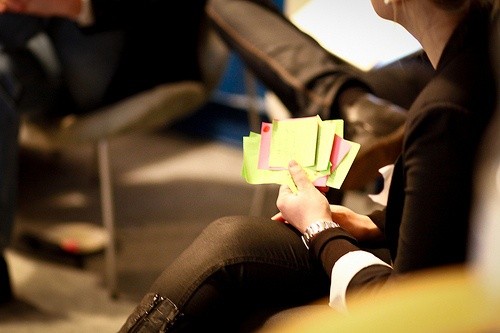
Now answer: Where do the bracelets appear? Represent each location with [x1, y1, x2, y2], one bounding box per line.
[300, 221, 340, 250]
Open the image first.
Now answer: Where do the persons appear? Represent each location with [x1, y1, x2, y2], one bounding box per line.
[1, 0, 205, 313]
[119, 0, 500, 333]
[204, 1, 436, 205]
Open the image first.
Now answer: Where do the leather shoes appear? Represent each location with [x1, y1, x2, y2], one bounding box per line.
[342, 92, 410, 193]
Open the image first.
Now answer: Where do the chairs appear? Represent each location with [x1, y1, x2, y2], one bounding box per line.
[19, 27, 232, 299]
